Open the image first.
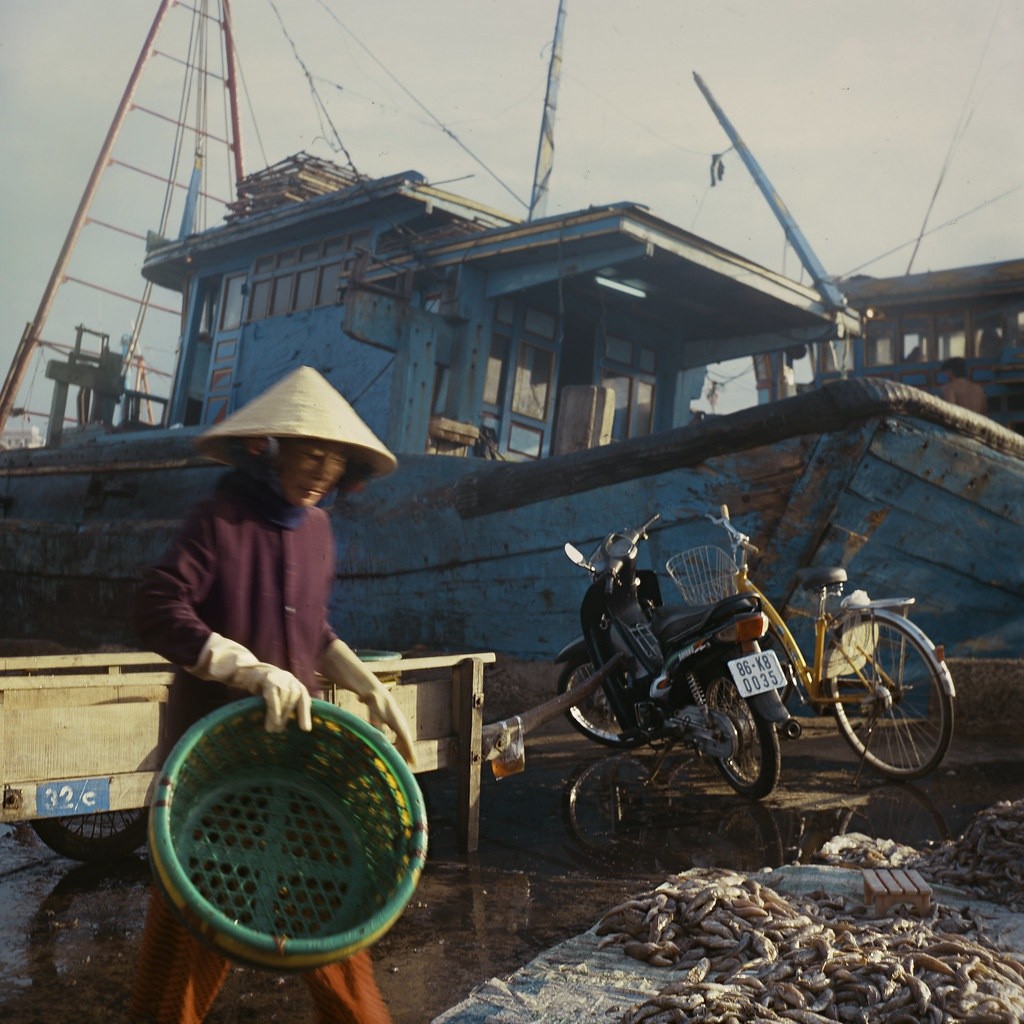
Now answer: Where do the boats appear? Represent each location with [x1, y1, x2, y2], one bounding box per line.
[2, 3, 1024, 774]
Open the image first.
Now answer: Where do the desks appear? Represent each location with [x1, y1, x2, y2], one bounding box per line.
[426, 417, 480, 457]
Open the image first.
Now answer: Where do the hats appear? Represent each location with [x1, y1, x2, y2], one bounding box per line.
[197, 366, 396, 489]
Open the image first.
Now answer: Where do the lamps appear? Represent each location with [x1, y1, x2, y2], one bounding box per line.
[595, 275, 647, 300]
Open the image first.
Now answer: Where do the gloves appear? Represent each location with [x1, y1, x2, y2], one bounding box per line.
[313, 640, 418, 767]
[181, 634, 312, 733]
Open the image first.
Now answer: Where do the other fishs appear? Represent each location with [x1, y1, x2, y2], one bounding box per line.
[596, 800, 1024, 1023]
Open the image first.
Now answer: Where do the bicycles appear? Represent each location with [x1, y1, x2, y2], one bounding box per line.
[664, 505, 956, 785]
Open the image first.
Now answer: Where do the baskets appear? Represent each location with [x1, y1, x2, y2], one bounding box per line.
[664, 545, 740, 607]
[147, 692, 427, 973]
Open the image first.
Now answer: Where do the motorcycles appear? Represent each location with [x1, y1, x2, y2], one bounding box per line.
[553, 510, 804, 801]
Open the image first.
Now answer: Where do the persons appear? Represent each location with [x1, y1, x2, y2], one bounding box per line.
[944, 357, 989, 416]
[145, 369, 416, 1023]
